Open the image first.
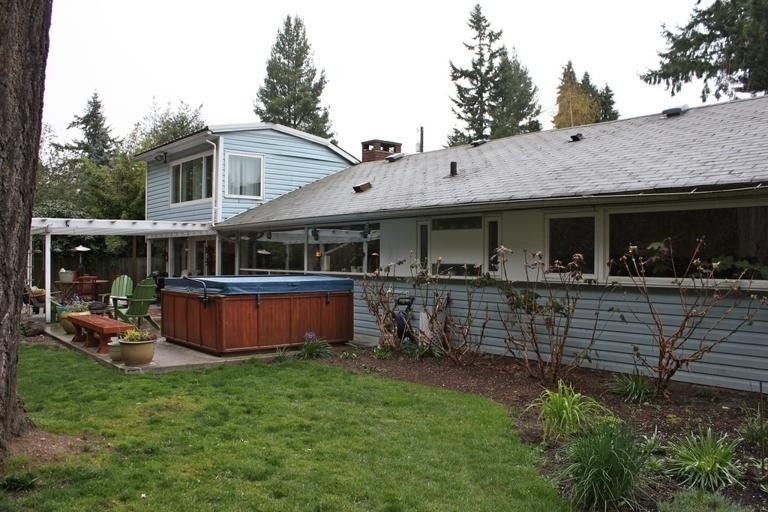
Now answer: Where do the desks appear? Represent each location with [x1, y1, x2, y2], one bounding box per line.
[88, 305, 115, 319]
[55, 279, 108, 300]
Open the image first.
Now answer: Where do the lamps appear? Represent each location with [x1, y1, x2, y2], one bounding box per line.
[71, 234, 92, 252]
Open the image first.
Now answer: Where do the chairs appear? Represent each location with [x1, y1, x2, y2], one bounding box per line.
[21, 281, 47, 315]
[93, 273, 134, 320]
[113, 277, 160, 333]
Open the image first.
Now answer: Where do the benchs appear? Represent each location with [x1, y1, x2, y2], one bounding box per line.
[67, 314, 136, 354]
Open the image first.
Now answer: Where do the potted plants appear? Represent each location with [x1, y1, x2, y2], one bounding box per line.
[58, 296, 91, 334]
[118, 327, 158, 365]
